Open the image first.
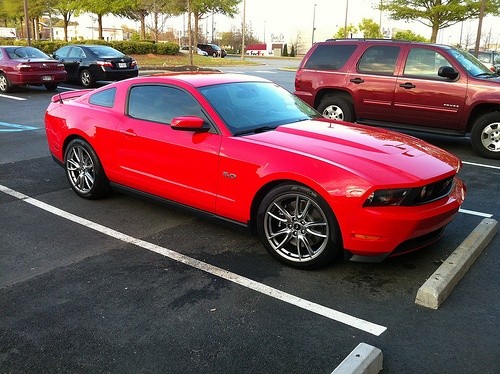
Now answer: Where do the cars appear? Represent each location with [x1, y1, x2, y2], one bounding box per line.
[0, 45, 68, 93]
[51, 43, 139, 89]
[179, 45, 208, 56]
[44, 70, 467, 272]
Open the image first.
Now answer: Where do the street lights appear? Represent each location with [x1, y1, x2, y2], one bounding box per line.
[312, 3, 317, 46]
[264, 21, 266, 44]
[448, 36, 452, 44]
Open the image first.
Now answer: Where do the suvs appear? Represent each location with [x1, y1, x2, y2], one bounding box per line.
[468, 48, 500, 75]
[292, 38, 500, 162]
[197, 43, 227, 58]
